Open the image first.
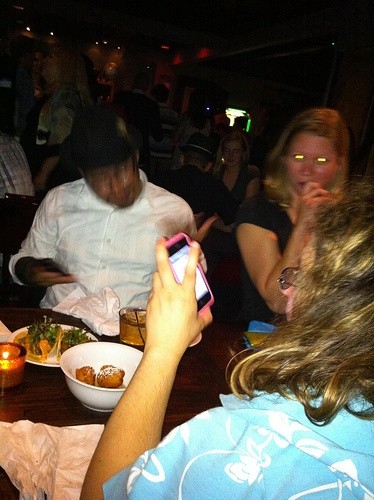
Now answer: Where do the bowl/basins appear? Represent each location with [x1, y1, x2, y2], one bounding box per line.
[60, 342, 143, 412]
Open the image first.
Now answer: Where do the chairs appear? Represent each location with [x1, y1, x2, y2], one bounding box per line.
[0, 193, 45, 290]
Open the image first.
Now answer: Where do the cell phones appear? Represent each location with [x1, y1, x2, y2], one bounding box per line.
[36, 257, 64, 277]
[165, 231, 215, 316]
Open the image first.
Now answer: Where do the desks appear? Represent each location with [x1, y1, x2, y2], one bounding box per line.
[0, 309, 254, 500]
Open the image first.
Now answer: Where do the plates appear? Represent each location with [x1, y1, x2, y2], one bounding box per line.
[7, 323, 99, 367]
[187, 332, 202, 347]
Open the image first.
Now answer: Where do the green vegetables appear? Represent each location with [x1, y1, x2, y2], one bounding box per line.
[27, 315, 92, 346]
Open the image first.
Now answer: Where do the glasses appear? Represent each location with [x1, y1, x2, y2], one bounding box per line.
[277, 265, 302, 291]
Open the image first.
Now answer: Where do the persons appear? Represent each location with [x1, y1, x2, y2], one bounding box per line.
[153, 130, 262, 275]
[0, 131, 34, 290]
[7, 118, 222, 335]
[78, 172, 373, 500]
[232, 109, 352, 327]
[0, 38, 208, 198]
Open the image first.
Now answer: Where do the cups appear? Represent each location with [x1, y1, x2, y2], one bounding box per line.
[0, 342, 27, 396]
[118, 306, 147, 351]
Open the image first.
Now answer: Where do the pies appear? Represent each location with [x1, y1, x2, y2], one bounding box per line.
[14, 325, 67, 363]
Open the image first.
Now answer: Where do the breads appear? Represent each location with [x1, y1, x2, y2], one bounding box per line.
[76, 365, 125, 388]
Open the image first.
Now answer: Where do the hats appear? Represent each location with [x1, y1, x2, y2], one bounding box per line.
[149, 83, 170, 95]
[63, 103, 143, 170]
